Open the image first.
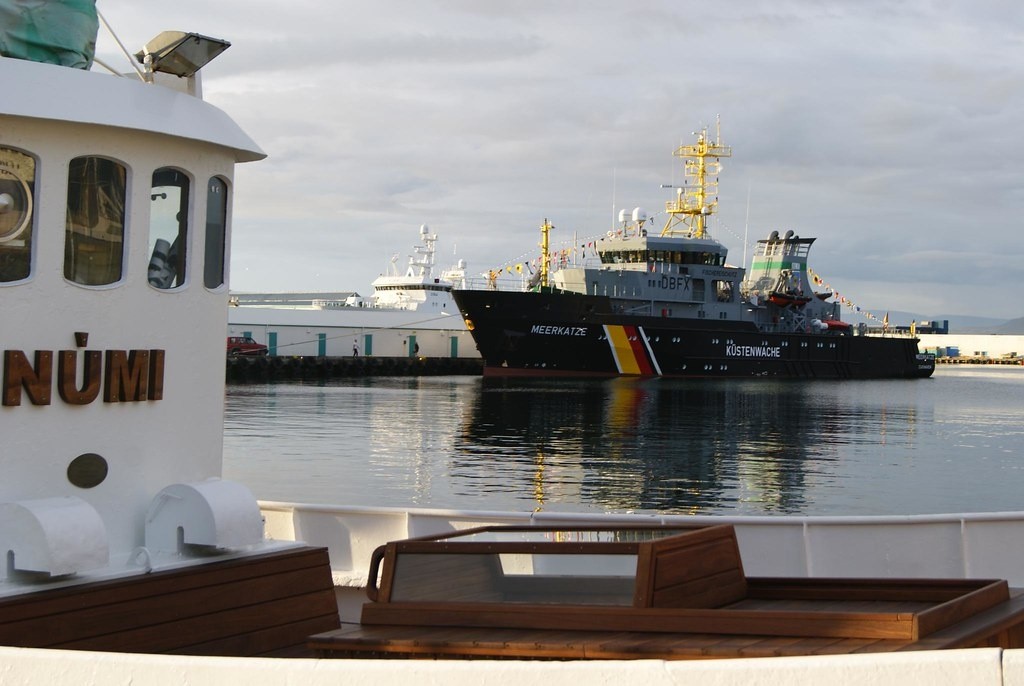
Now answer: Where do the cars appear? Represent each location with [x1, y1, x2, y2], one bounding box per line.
[227, 335, 268, 358]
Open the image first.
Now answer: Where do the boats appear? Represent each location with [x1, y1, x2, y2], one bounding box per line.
[769, 292, 812, 306]
[451, 107, 935, 384]
[227, 221, 483, 360]
[822, 320, 850, 327]
[1, 0, 1024, 686]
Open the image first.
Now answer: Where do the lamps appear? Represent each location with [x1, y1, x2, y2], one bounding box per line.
[133, 31, 231, 100]
